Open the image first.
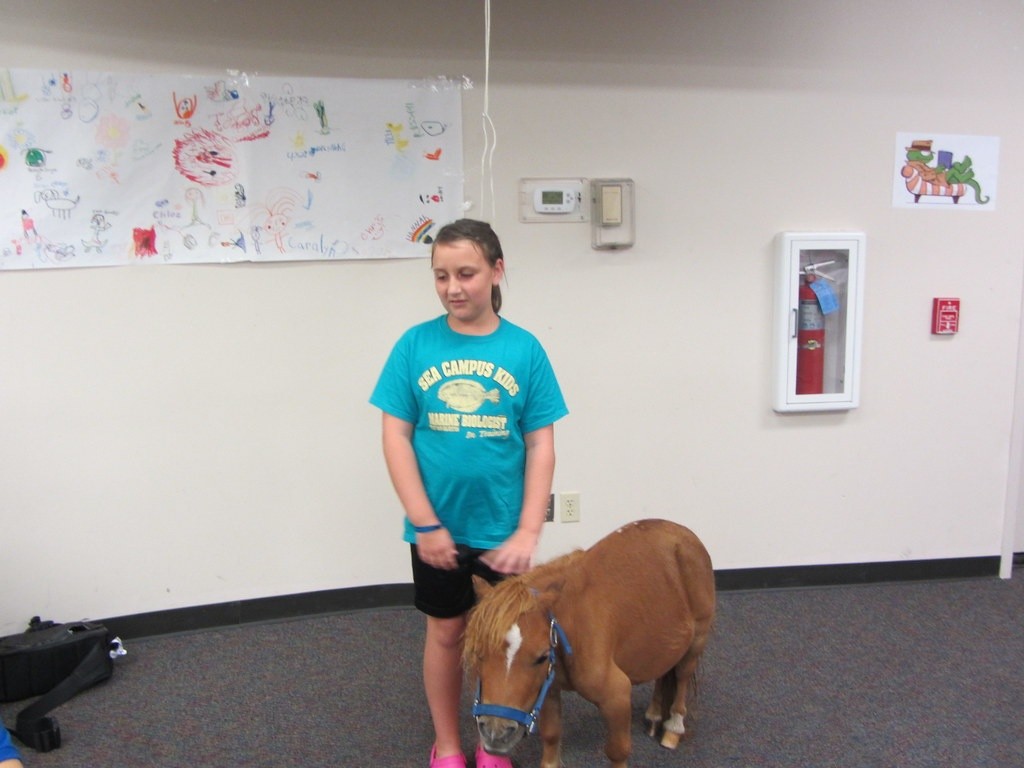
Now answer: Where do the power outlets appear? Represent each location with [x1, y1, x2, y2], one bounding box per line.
[560, 494, 580, 522]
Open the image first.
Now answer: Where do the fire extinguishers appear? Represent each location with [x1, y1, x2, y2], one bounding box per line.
[796, 260, 838, 393]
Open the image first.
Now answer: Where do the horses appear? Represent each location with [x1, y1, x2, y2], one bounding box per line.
[468, 516, 718, 768]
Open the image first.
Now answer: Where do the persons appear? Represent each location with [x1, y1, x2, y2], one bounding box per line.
[366, 218, 570, 768]
[0, 722, 21, 768]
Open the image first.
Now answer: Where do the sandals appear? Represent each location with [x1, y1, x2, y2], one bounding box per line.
[430, 743, 467, 768]
[476, 742, 512, 768]
